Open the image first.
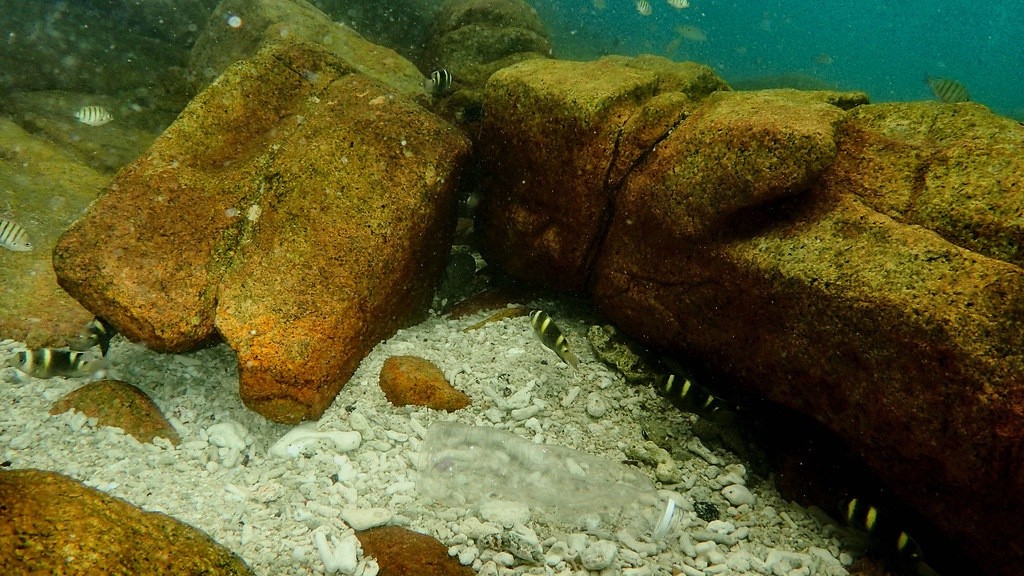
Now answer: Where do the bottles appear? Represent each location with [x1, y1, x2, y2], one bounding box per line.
[414, 421, 684, 545]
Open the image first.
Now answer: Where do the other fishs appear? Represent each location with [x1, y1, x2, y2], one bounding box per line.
[1, 105, 117, 380]
[420, 0, 971, 563]
